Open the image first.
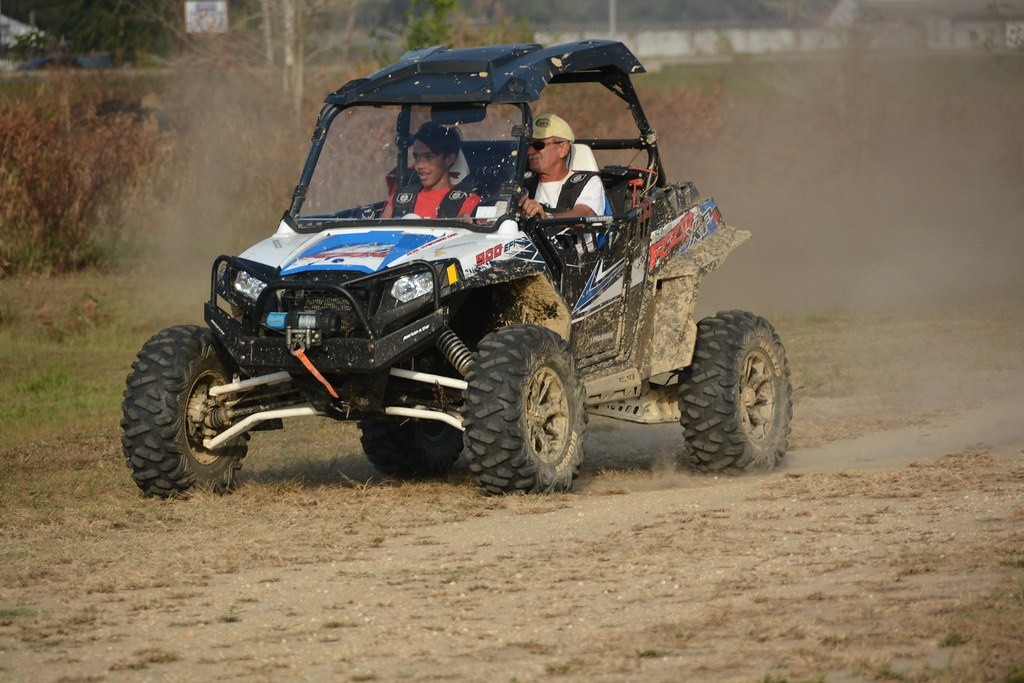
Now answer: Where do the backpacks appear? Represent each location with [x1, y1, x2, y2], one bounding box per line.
[523, 171, 618, 252]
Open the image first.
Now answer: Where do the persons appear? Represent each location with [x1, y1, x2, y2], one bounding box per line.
[518, 112, 606, 252]
[381, 121, 482, 219]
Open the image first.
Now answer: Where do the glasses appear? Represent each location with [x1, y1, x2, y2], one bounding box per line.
[530, 141, 564, 150]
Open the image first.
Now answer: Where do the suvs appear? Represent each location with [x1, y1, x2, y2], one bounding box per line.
[120, 39, 795, 503]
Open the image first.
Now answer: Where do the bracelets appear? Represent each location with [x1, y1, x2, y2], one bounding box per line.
[545, 212, 557, 219]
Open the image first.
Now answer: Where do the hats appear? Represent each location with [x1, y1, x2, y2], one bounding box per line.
[532, 113, 574, 142]
